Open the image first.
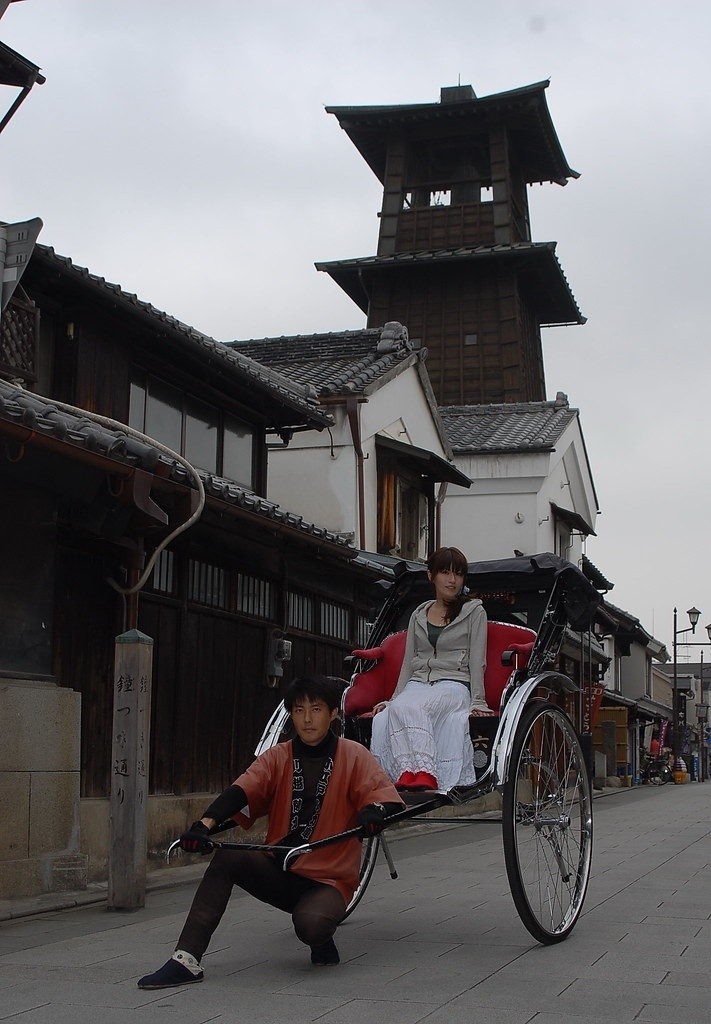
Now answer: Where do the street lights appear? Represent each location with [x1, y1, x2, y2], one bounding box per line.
[670, 606, 711, 762]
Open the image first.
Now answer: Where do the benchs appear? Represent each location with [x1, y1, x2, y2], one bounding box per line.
[341, 619, 540, 730]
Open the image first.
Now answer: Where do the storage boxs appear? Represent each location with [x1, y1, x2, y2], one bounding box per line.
[620, 775, 633, 787]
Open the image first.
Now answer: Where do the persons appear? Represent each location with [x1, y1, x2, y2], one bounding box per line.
[137, 674, 406, 988]
[366, 546, 495, 791]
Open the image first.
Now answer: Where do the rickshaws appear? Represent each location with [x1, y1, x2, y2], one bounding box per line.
[165, 552, 605, 945]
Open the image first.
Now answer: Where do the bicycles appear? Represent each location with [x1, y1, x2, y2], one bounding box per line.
[640, 746, 671, 786]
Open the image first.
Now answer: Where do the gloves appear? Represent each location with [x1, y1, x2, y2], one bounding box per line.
[179, 820, 213, 856]
[355, 806, 382, 842]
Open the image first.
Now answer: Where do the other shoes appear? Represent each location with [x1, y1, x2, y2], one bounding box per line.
[137, 958, 203, 989]
[394, 771, 415, 790]
[410, 771, 438, 790]
[310, 936, 339, 966]
